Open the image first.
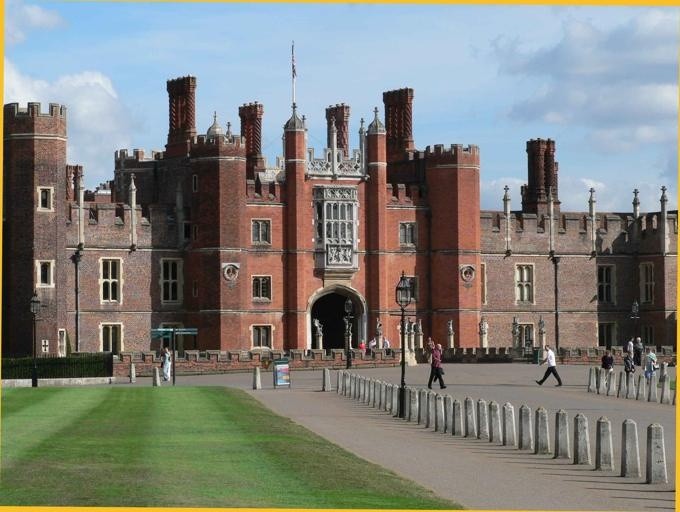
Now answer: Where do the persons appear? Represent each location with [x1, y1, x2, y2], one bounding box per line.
[634, 337, 643, 366]
[534, 345, 563, 387]
[382, 335, 390, 357]
[623, 350, 635, 387]
[424, 336, 435, 364]
[369, 336, 378, 358]
[427, 343, 447, 389]
[161, 346, 171, 384]
[600, 349, 615, 387]
[357, 338, 366, 358]
[626, 335, 633, 362]
[643, 347, 658, 388]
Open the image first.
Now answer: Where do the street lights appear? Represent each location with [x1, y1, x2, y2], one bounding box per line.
[393, 269, 417, 420]
[629, 298, 643, 375]
[30, 285, 44, 391]
[151, 327, 198, 338]
[343, 295, 357, 372]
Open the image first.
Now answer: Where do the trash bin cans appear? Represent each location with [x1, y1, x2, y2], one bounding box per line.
[532, 347, 541, 364]
